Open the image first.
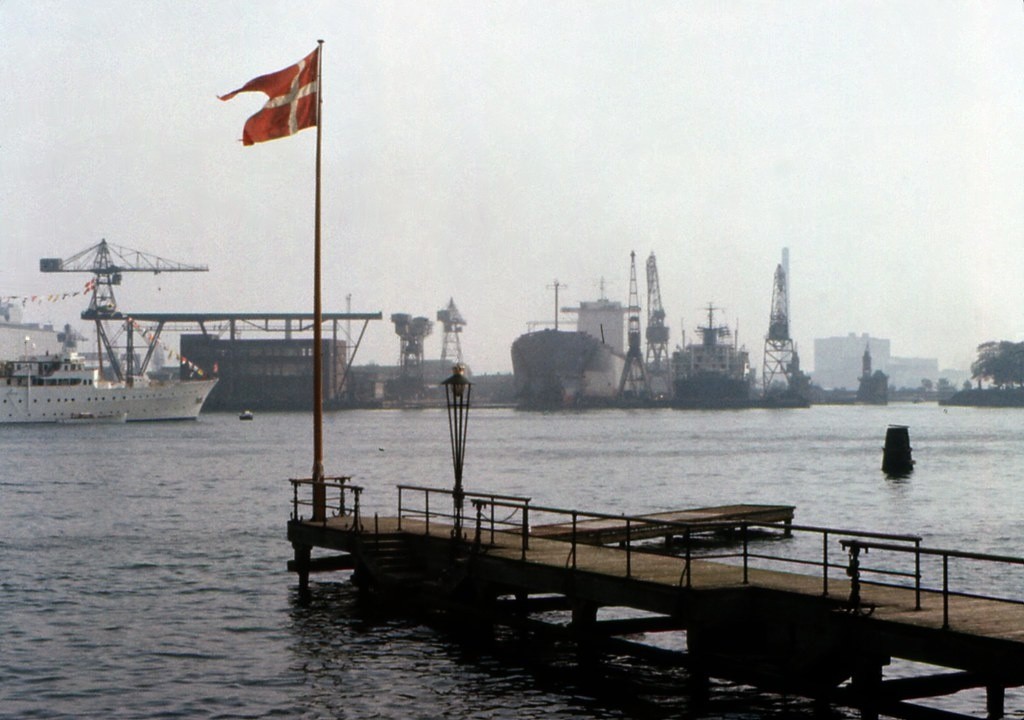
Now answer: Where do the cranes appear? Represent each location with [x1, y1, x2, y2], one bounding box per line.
[37, 239, 212, 313]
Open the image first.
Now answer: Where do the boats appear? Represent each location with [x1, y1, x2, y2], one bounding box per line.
[882, 423, 914, 474]
[238, 411, 253, 421]
[0, 239, 222, 423]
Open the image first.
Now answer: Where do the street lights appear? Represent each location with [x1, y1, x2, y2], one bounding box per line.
[439, 361, 476, 537]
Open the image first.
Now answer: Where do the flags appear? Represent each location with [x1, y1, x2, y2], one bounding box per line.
[216, 46, 318, 143]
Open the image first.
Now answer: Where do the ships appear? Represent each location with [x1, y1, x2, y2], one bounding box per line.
[509, 277, 674, 407]
